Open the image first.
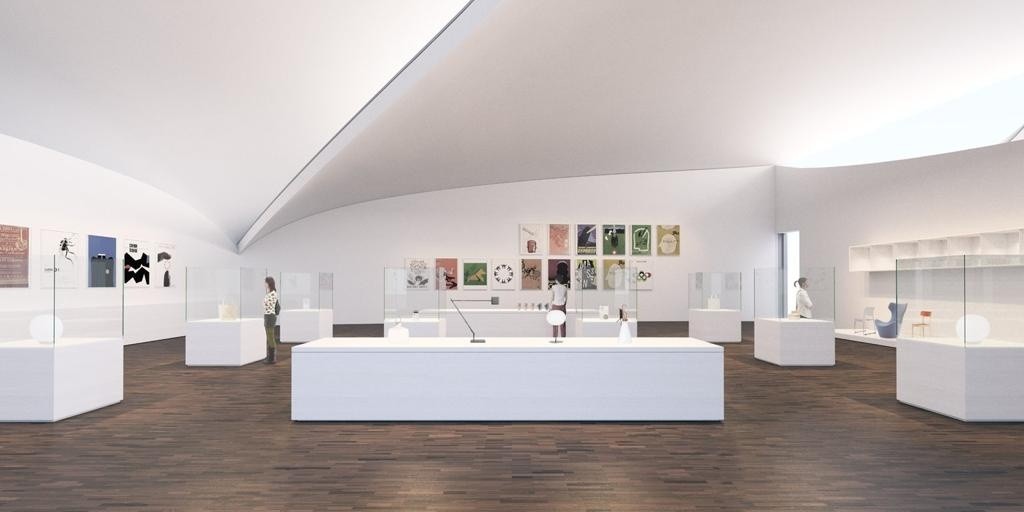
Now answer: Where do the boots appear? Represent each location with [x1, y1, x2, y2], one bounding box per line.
[263, 348, 277, 363]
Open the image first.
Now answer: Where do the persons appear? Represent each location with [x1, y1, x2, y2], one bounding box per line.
[617, 307, 631, 337]
[793, 278, 813, 319]
[261, 276, 281, 365]
[548, 275, 567, 338]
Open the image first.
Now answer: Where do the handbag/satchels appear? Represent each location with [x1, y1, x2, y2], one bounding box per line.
[275, 300, 281, 315]
